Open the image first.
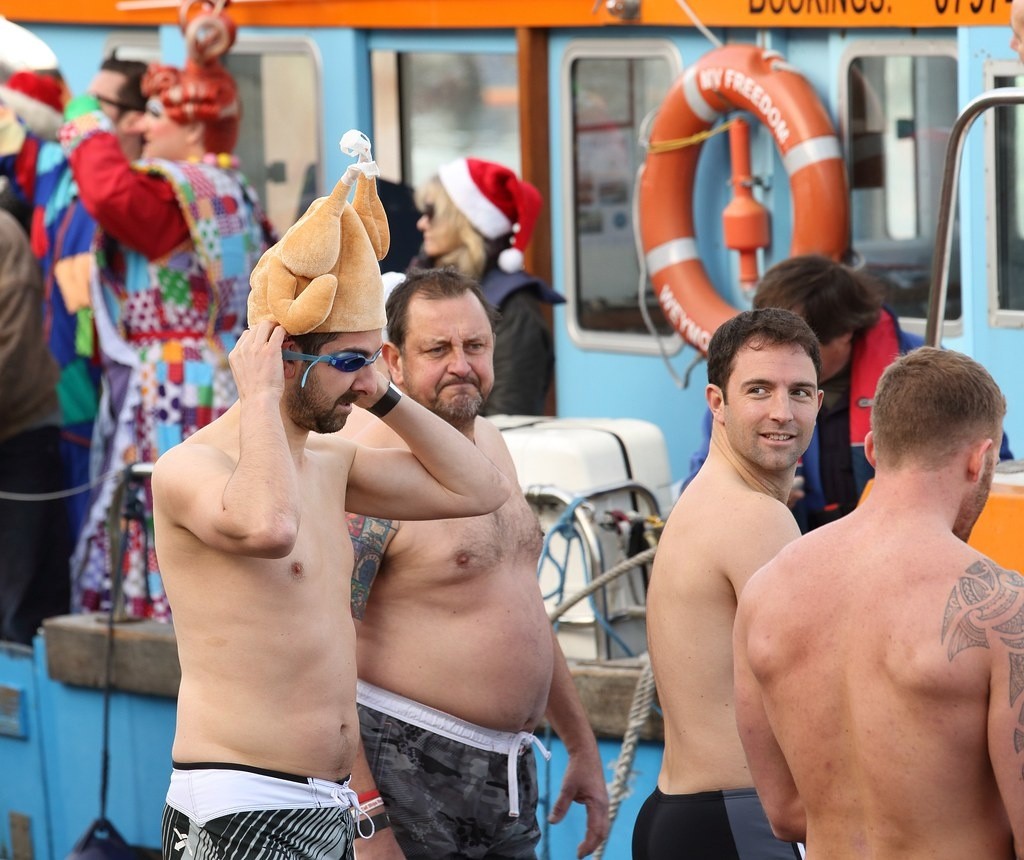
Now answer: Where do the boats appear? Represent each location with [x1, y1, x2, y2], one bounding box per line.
[0, 1, 1022, 860]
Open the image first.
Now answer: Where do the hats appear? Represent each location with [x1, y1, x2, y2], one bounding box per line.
[438, 158, 542, 273]
[247, 131, 390, 337]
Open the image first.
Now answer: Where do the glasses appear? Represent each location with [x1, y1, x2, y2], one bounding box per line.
[423, 205, 435, 222]
[282, 347, 383, 387]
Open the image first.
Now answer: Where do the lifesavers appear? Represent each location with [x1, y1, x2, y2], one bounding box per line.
[632, 46, 860, 366]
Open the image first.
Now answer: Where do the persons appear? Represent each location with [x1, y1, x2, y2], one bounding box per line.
[681, 254, 1014, 538]
[0, 1, 277, 618]
[632, 307, 824, 860]
[344, 268, 610, 860]
[411, 158, 567, 414]
[732, 345, 1024, 860]
[151, 127, 511, 860]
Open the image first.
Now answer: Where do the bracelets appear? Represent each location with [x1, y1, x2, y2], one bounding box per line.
[350, 789, 391, 839]
[365, 380, 403, 418]
